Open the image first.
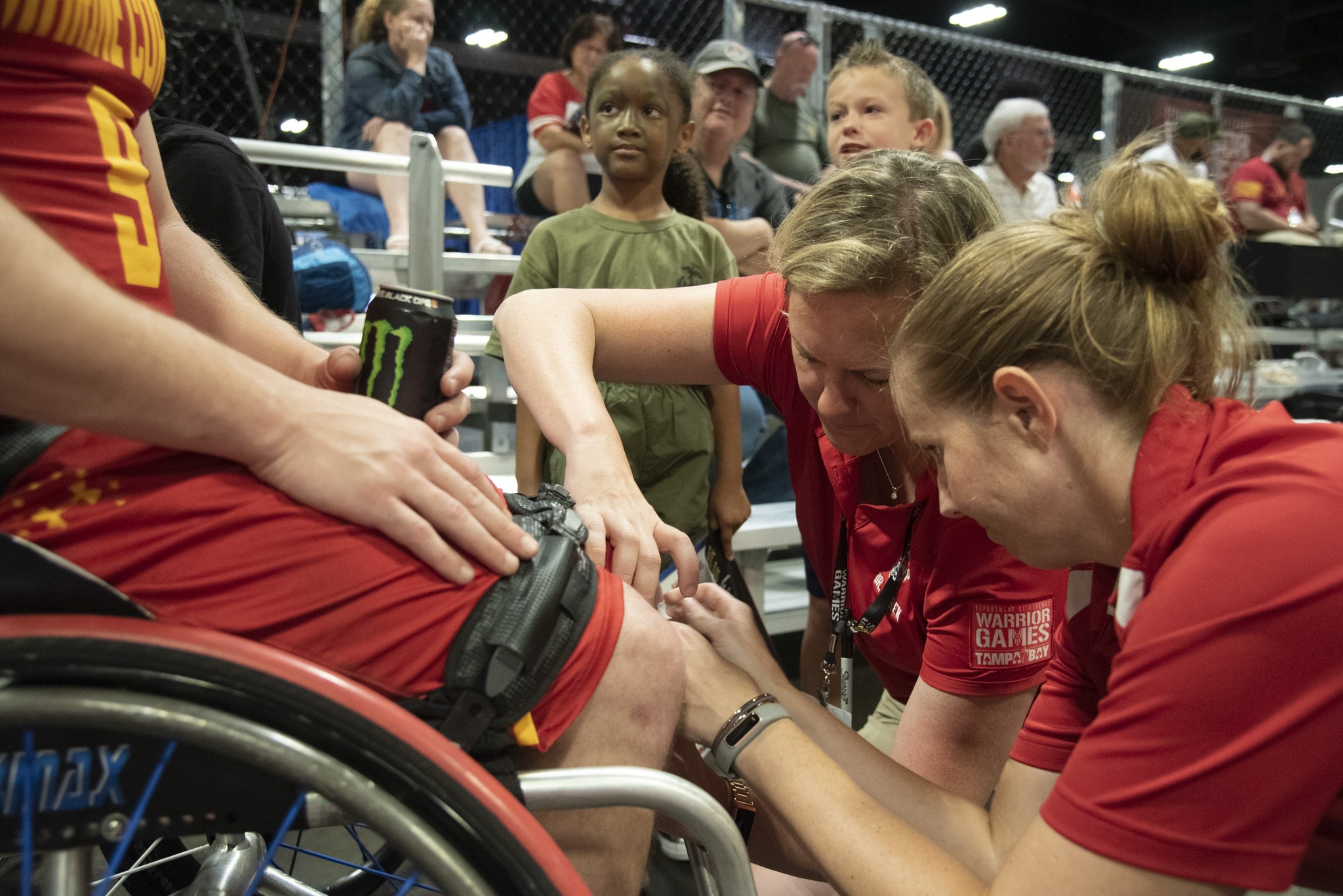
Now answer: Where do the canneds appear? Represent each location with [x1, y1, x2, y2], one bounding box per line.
[354, 284, 458, 424]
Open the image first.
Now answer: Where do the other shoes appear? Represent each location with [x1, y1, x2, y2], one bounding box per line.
[383, 235, 410, 251]
[471, 238, 513, 255]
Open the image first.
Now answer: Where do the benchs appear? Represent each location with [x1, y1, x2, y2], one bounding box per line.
[270, 193, 808, 636]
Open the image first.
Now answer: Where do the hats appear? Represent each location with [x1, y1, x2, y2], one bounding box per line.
[688, 39, 764, 87]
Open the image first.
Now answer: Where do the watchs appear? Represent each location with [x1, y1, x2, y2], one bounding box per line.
[694, 692, 793, 777]
[721, 777, 756, 849]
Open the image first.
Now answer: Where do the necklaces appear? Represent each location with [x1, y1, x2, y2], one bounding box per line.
[876, 449, 926, 500]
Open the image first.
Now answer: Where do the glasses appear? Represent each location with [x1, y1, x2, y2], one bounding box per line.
[782, 37, 819, 48]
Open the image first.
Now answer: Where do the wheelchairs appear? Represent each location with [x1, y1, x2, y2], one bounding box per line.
[1, 415, 769, 896]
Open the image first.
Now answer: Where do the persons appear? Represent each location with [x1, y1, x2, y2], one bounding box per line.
[148, 107, 303, 337]
[970, 97, 1060, 227]
[1219, 122, 1324, 239]
[1130, 110, 1217, 186]
[330, 0, 513, 258]
[492, 11, 1343, 896]
[0, 0, 684, 896]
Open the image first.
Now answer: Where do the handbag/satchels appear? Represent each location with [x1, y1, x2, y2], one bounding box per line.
[291, 237, 372, 331]
